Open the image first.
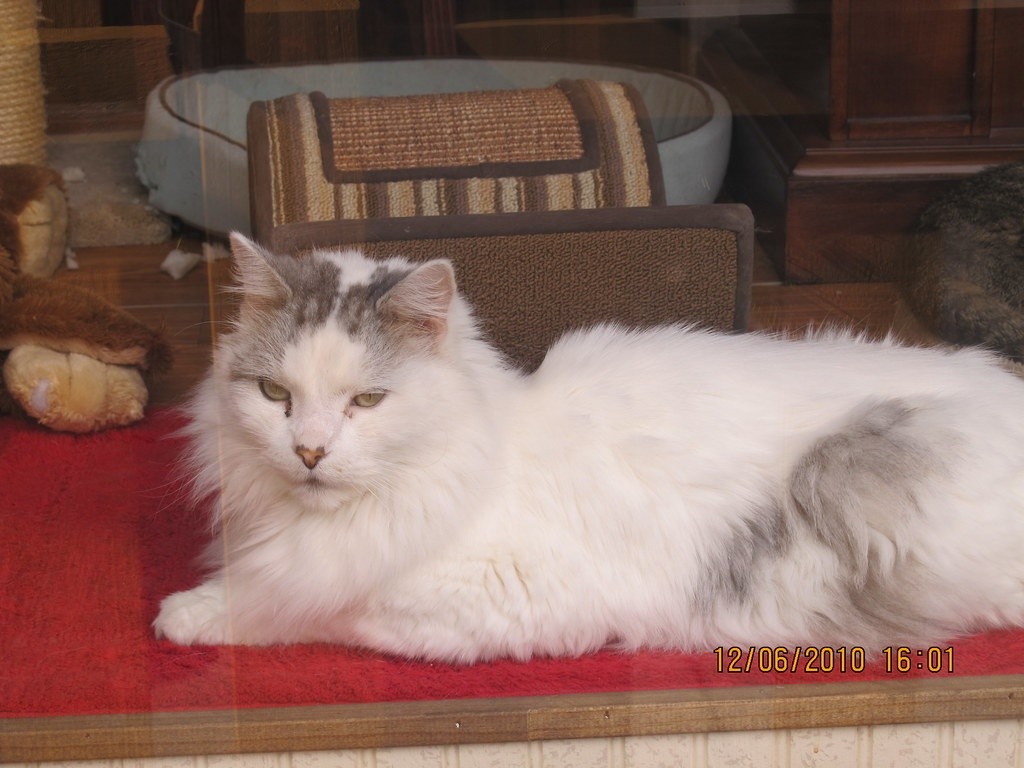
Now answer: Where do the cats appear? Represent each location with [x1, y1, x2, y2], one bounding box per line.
[146, 229, 1024, 664]
[899, 164, 1024, 365]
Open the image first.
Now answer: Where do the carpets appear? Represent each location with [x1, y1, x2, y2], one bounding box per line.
[1, 402, 1023, 719]
